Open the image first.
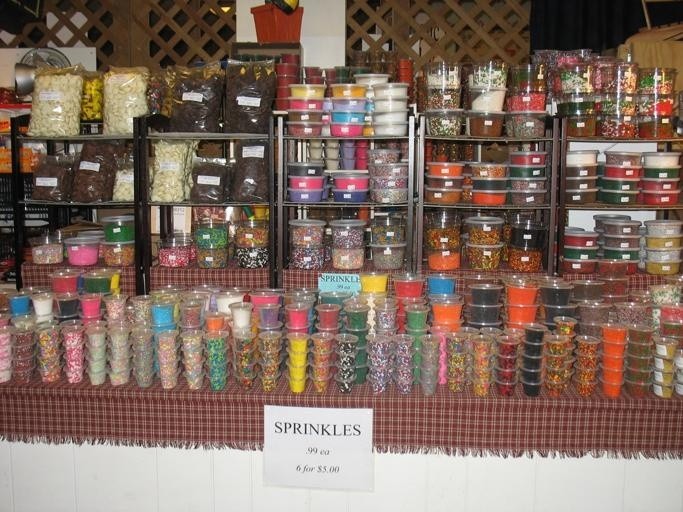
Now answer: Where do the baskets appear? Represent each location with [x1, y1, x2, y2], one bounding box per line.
[0, 172, 92, 282]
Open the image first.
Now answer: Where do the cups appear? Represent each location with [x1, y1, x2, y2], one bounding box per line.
[287, 271, 683, 399]
[1, 284, 285, 393]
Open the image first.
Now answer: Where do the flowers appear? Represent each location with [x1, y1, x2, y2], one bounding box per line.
[264, 0, 299, 13]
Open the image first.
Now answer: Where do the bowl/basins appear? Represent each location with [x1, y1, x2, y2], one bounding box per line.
[0, 206, 274, 269]
[29, 213, 134, 267]
[277, 50, 681, 274]
[50, 268, 124, 295]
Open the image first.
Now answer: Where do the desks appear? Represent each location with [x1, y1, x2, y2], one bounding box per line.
[1, 375, 683, 459]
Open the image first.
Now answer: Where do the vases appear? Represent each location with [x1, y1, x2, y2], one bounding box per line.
[251, 4, 304, 45]
